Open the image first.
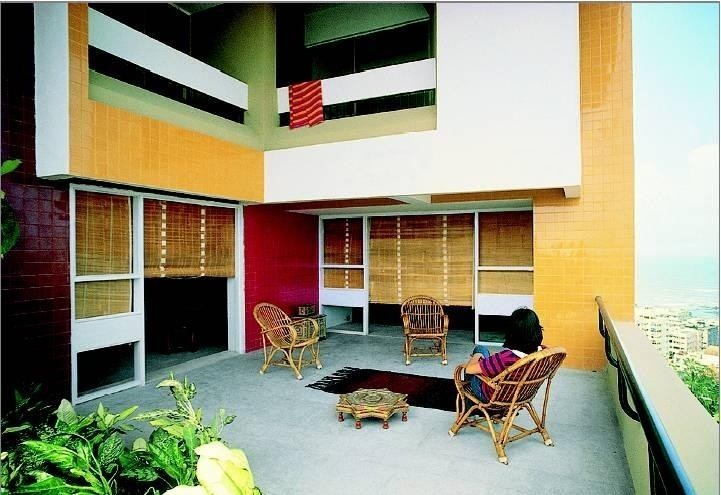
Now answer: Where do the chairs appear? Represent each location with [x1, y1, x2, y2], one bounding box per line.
[253, 303, 322, 380]
[400, 295, 449, 366]
[448, 347, 567, 464]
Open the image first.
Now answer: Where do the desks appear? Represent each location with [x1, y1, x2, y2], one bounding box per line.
[335, 388, 410, 429]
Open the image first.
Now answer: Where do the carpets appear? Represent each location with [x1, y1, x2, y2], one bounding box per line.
[305, 367, 523, 417]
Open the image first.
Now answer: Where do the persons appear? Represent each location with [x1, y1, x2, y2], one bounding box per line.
[456, 307, 552, 429]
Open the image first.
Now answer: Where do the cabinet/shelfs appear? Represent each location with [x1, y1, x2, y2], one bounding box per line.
[291, 314, 327, 341]
